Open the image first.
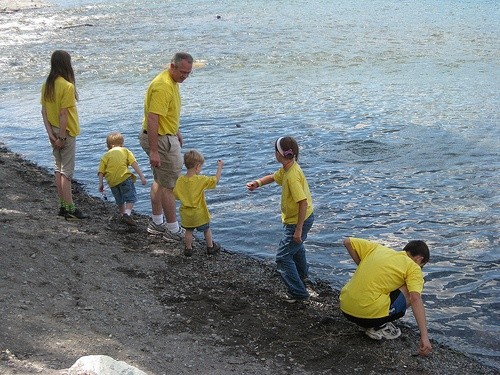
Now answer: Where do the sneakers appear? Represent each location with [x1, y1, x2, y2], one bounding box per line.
[365, 321, 402, 340]
[147, 221, 195, 243]
[281, 293, 310, 302]
[58, 201, 90, 220]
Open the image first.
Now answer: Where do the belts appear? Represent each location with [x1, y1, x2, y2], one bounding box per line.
[143, 130, 171, 136]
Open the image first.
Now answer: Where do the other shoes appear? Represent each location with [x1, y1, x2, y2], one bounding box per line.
[120, 213, 137, 226]
[183, 244, 193, 257]
[207, 242, 221, 255]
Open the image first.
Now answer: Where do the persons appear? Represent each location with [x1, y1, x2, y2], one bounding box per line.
[246, 136, 315, 303]
[137, 53, 196, 243]
[97, 132, 148, 226]
[339, 237, 432, 356]
[40, 50, 90, 221]
[172, 150, 224, 257]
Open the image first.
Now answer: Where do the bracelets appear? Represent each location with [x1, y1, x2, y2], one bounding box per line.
[58, 136, 66, 141]
